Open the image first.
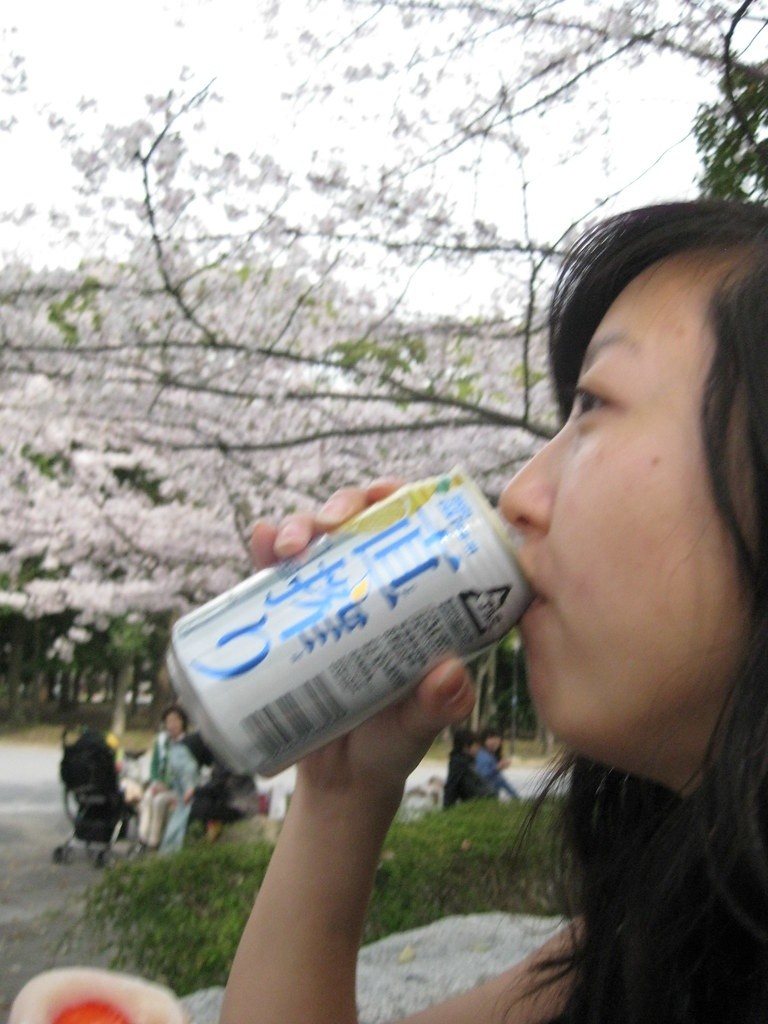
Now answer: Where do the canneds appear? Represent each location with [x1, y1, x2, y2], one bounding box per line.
[165, 466, 535, 777]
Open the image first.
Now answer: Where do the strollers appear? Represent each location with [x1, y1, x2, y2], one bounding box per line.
[51, 722, 142, 870]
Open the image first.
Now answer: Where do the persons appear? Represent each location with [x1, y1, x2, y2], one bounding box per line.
[209, 197, 765, 1024]
[97, 704, 259, 865]
[477, 722, 525, 803]
[441, 726, 497, 815]
